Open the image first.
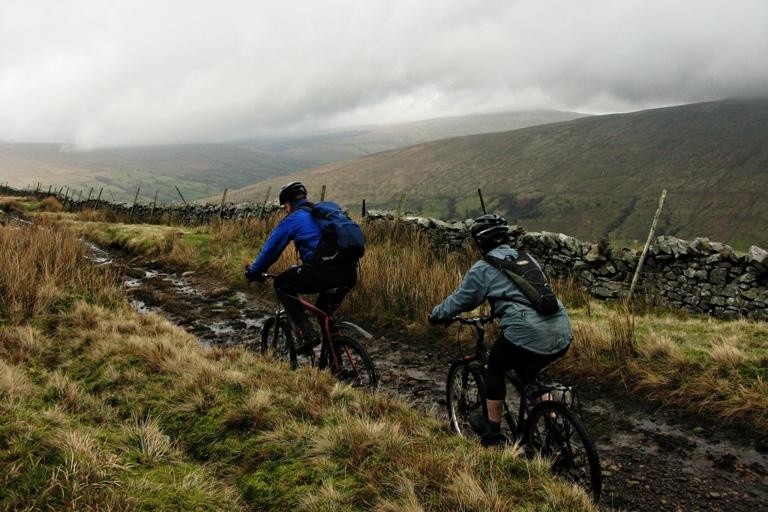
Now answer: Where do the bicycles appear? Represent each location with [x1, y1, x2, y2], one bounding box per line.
[427, 309, 602, 507]
[244, 264, 381, 391]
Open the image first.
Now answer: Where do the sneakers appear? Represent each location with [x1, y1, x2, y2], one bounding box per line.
[295, 335, 321, 353]
[468, 415, 501, 445]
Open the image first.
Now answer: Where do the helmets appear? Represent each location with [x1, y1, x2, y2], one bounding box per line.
[471, 214, 508, 239]
[279, 181, 306, 204]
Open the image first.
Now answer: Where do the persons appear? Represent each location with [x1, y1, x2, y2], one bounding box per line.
[430, 214, 573, 448]
[247, 181, 357, 353]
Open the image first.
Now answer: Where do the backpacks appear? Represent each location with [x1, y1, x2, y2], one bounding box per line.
[483, 246, 559, 317]
[298, 202, 365, 253]
